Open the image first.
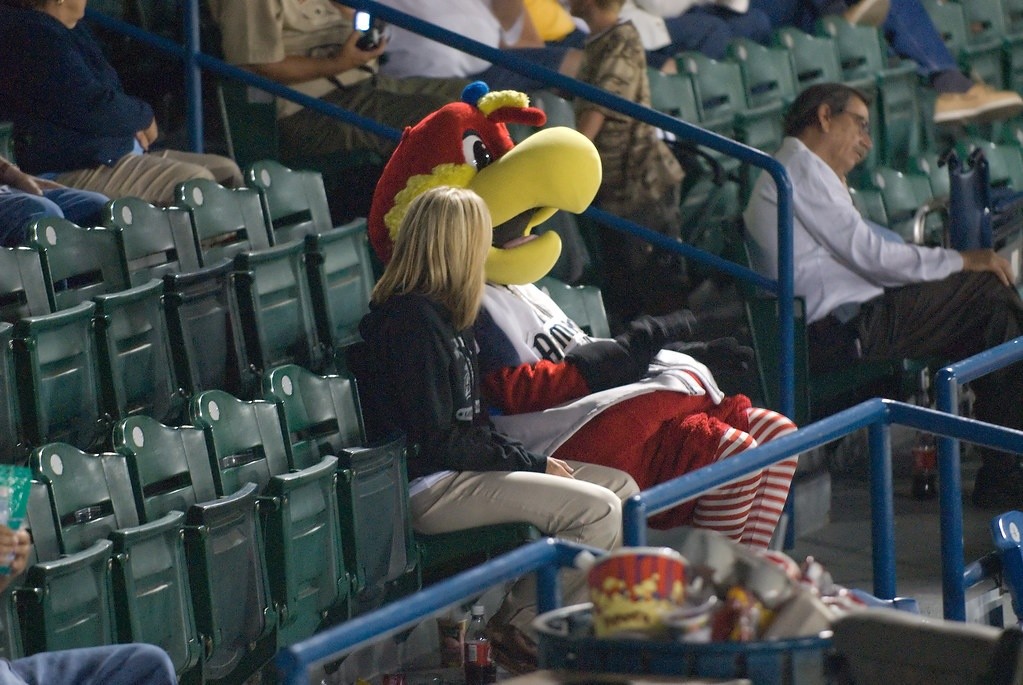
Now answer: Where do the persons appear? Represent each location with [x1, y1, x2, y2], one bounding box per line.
[0, 156, 111, 289]
[743, 83, 1023, 511]
[0, 0, 247, 252]
[0, 525, 179, 685]
[205, 0, 479, 160]
[546, 1, 684, 302]
[365, 0, 1023, 129]
[359, 188, 641, 676]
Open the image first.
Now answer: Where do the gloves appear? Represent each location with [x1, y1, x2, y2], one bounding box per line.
[565, 309, 698, 392]
[661, 337, 754, 384]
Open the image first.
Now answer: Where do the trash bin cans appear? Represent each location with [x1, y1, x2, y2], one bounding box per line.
[530, 595, 835, 685]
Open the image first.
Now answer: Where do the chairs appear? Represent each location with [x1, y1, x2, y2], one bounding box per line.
[993, 510, 1022, 624]
[508, 0, 1023, 397]
[830, 590, 1023, 685]
[0, 121, 613, 685]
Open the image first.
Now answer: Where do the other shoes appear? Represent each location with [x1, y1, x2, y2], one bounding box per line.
[973, 470, 1023, 510]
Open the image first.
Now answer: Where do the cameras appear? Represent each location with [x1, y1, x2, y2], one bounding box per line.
[636, 309, 699, 355]
[354, 9, 391, 52]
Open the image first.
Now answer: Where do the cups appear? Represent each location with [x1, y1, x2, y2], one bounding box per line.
[437, 616, 468, 669]
[666, 594, 719, 642]
[584, 552, 689, 640]
[762, 549, 802, 580]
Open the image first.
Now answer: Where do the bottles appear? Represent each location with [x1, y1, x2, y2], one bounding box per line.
[912, 430, 938, 501]
[464, 606, 493, 685]
[0, 486, 15, 577]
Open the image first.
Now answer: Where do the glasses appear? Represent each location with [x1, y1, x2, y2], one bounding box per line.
[832, 109, 870, 135]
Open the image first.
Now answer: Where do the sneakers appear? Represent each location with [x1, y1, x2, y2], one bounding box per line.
[487, 623, 540, 676]
[828, 0, 890, 38]
[933, 84, 1023, 125]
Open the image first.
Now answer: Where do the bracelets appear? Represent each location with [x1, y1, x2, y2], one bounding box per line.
[0, 162, 17, 174]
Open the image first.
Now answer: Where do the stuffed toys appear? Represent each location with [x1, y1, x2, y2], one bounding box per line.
[368, 81, 799, 578]
